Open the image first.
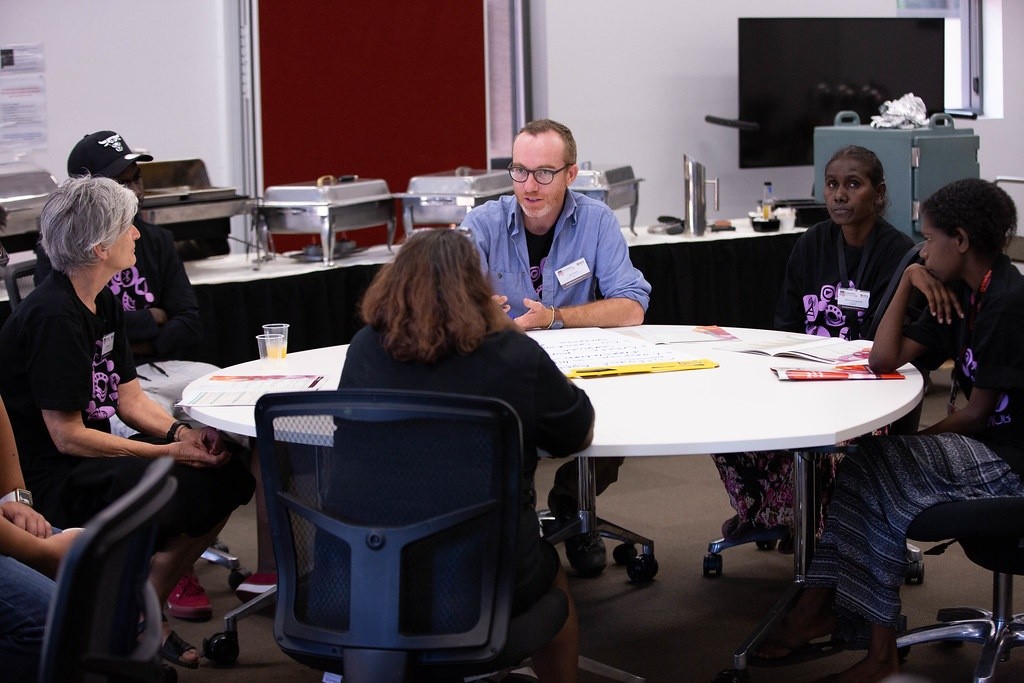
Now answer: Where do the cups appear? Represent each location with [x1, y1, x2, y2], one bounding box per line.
[263, 324, 289, 360]
[777, 216, 795, 230]
[256, 334, 284, 371]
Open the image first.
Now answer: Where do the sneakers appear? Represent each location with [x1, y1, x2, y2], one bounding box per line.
[235, 571, 279, 601]
[547, 485, 607, 576]
[166, 576, 212, 621]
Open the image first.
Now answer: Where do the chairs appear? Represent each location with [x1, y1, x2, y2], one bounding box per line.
[4, 259, 1024, 683]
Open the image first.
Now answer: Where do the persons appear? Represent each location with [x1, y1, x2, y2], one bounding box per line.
[0, 169, 234, 667]
[802, 177, 1024, 683]
[0, 204, 89, 683]
[709, 144, 922, 555]
[313, 226, 581, 683]
[33, 130, 255, 550]
[456, 119, 651, 575]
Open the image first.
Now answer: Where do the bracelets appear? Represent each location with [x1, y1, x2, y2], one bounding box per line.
[174, 425, 186, 441]
[543, 305, 555, 329]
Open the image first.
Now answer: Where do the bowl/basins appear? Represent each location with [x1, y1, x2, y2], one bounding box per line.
[752, 217, 780, 232]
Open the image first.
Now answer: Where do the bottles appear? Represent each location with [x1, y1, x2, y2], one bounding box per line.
[764, 182, 773, 217]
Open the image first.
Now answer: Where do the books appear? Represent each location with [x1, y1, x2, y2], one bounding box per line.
[712, 332, 874, 364]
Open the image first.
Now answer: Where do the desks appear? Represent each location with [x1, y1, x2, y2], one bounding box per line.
[181, 325, 928, 682]
[0, 217, 809, 476]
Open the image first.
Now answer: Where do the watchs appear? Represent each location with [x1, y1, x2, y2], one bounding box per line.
[167, 421, 192, 442]
[551, 308, 564, 329]
[0, 488, 34, 506]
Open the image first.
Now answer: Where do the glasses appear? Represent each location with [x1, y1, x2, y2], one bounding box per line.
[116, 169, 143, 187]
[507, 161, 573, 185]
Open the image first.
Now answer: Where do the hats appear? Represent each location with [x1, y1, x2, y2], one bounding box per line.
[67, 131, 152, 180]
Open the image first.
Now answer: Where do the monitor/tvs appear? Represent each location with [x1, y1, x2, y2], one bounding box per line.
[737, 17, 945, 168]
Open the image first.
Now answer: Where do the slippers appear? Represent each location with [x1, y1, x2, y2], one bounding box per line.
[156, 630, 201, 670]
[751, 639, 844, 665]
